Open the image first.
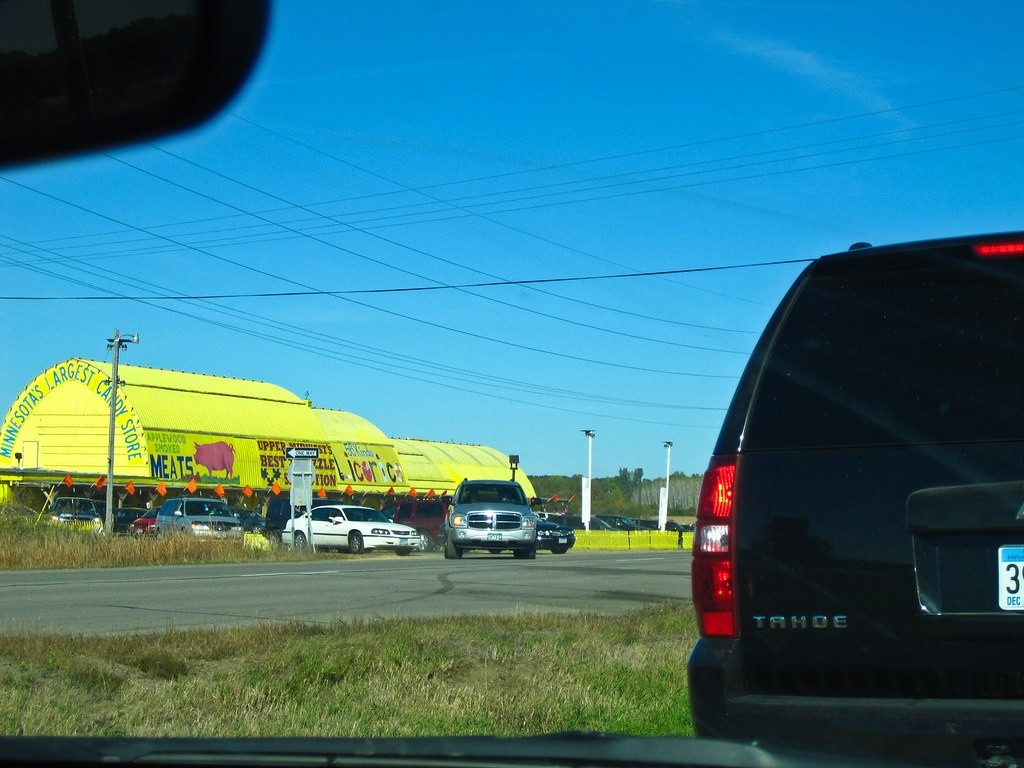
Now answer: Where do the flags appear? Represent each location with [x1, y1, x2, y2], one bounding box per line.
[317, 488, 326, 498]
[571, 494, 577, 500]
[125, 481, 135, 495]
[408, 487, 417, 497]
[386, 486, 394, 493]
[272, 483, 282, 495]
[440, 490, 447, 496]
[96, 476, 106, 488]
[529, 497, 533, 505]
[345, 485, 353, 496]
[187, 479, 197, 493]
[242, 485, 253, 497]
[552, 495, 558, 502]
[62, 475, 73, 487]
[215, 484, 225, 497]
[157, 482, 166, 496]
[427, 489, 435, 498]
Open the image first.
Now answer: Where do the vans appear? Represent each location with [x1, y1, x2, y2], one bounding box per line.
[265, 498, 345, 544]
[156, 497, 244, 547]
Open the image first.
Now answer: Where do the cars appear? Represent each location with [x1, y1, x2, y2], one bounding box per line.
[113, 507, 150, 534]
[47, 497, 103, 534]
[128, 506, 162, 538]
[230, 509, 266, 535]
[533, 513, 576, 555]
[534, 511, 694, 541]
[281, 505, 420, 556]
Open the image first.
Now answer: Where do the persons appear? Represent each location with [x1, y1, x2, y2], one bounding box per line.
[365, 512, 374, 520]
[336, 510, 342, 517]
[461, 486, 481, 503]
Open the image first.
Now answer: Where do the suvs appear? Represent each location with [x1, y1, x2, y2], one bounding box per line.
[443, 478, 539, 559]
[393, 501, 451, 552]
[686, 226, 1023, 768]
